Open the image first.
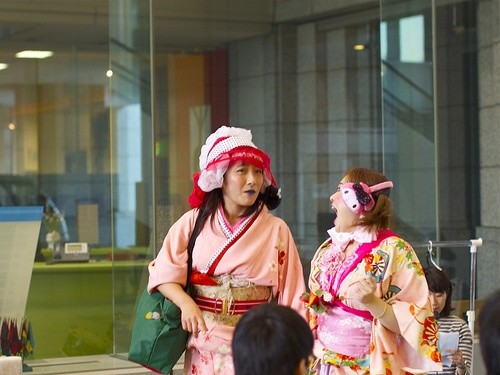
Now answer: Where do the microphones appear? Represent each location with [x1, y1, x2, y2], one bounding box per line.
[434, 311, 440, 320]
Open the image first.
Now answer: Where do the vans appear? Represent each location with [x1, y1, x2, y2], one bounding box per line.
[0, 172, 113, 250]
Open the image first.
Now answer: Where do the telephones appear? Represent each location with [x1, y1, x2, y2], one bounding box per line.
[52, 241, 90, 262]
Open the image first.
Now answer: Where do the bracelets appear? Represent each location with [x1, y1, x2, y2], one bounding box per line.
[457, 363, 466, 369]
[370, 301, 387, 318]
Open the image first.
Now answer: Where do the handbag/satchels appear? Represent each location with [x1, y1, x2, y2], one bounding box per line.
[127, 287, 189, 375]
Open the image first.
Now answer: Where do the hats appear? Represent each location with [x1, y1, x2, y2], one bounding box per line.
[189, 126, 281, 210]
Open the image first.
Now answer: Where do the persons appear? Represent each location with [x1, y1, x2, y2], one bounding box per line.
[478, 292, 500, 375]
[148, 126, 308, 375]
[233, 303, 314, 375]
[420, 265, 473, 375]
[302, 168, 443, 375]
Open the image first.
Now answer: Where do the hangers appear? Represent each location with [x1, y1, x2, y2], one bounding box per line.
[424, 238, 443, 272]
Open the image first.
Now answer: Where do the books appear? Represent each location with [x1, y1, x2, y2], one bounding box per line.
[436, 331, 459, 366]
